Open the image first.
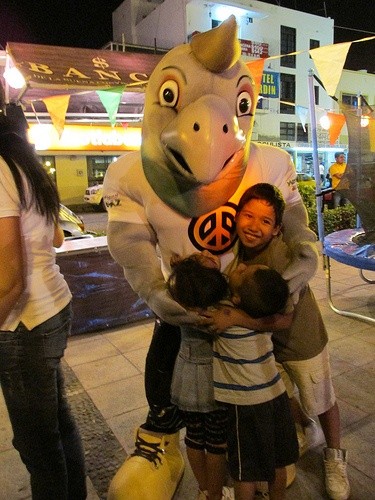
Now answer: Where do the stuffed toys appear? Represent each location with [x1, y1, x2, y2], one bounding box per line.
[102, 10, 357, 500]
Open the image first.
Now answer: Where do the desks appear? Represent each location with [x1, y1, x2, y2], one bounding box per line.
[321, 225, 375, 323]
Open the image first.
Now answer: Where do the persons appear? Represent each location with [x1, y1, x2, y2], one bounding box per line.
[165, 248, 232, 500]
[319, 162, 332, 189]
[329, 152, 353, 212]
[0, 83, 88, 500]
[194, 181, 353, 500]
[182, 260, 301, 500]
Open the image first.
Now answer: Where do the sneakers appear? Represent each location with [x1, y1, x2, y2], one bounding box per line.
[321, 446, 351, 500]
[294, 416, 326, 457]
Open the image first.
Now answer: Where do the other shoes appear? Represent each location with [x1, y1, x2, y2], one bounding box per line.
[198, 486, 234, 500]
[285, 463, 296, 488]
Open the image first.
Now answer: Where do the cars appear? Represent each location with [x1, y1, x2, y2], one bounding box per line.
[82, 184, 108, 212]
